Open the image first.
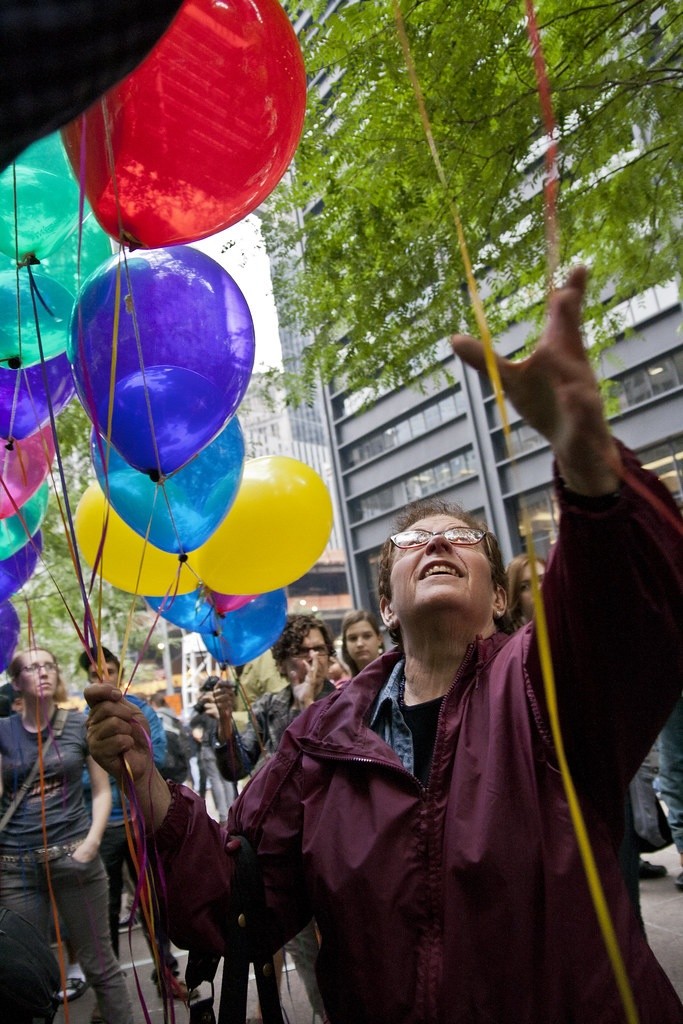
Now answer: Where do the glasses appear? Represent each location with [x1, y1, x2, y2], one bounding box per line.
[16, 662, 58, 675]
[385, 526, 494, 573]
[288, 645, 330, 658]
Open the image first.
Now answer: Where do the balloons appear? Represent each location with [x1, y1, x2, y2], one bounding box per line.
[0, 0, 184, 174]
[61, 0, 308, 254]
[0, 136, 335, 675]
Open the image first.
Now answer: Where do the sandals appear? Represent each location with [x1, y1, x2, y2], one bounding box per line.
[58, 978, 90, 1002]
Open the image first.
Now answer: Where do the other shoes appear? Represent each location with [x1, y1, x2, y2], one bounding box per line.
[639, 859, 666, 877]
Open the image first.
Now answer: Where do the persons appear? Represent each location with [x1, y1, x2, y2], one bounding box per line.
[0, 265, 683, 1024]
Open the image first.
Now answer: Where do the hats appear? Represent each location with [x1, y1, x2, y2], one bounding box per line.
[79, 646, 115, 669]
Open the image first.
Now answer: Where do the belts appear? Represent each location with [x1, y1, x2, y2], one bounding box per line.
[1, 838, 84, 864]
